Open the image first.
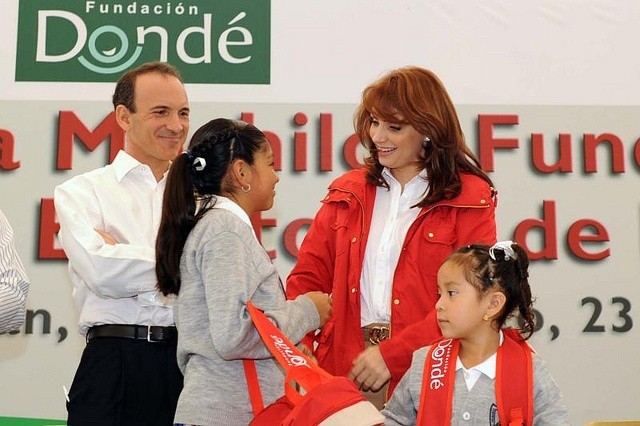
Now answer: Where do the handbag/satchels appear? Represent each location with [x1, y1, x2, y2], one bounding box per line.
[243, 301, 387, 426]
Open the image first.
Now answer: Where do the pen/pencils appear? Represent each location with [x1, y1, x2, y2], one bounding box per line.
[60, 383, 71, 405]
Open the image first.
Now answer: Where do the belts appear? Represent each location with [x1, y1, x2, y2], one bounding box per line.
[87, 324, 179, 344]
[362, 321, 391, 345]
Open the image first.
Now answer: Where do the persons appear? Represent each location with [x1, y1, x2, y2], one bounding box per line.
[155, 118, 331, 426]
[377, 240, 572, 426]
[286, 66, 498, 411]
[55, 61, 205, 426]
[0, 209, 30, 332]
